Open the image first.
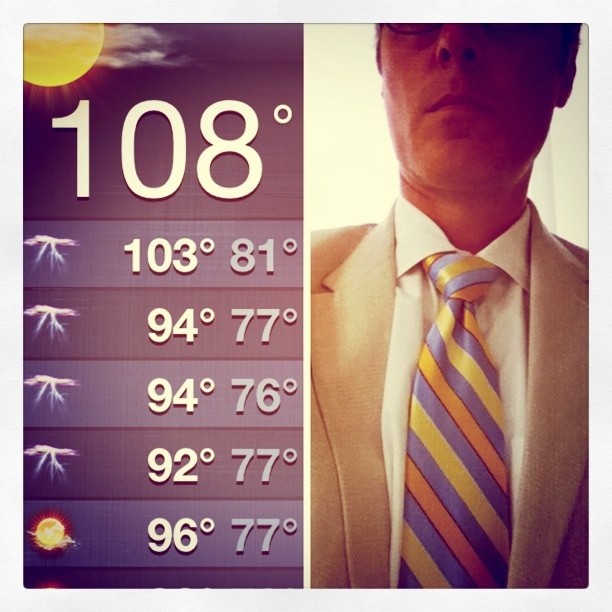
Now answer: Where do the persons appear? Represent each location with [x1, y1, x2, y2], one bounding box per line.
[306, 23, 589, 588]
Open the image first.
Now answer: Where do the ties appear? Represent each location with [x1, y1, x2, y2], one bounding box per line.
[397, 252, 512, 589]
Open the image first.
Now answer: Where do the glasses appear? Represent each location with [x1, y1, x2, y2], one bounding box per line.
[386, 22, 566, 39]
